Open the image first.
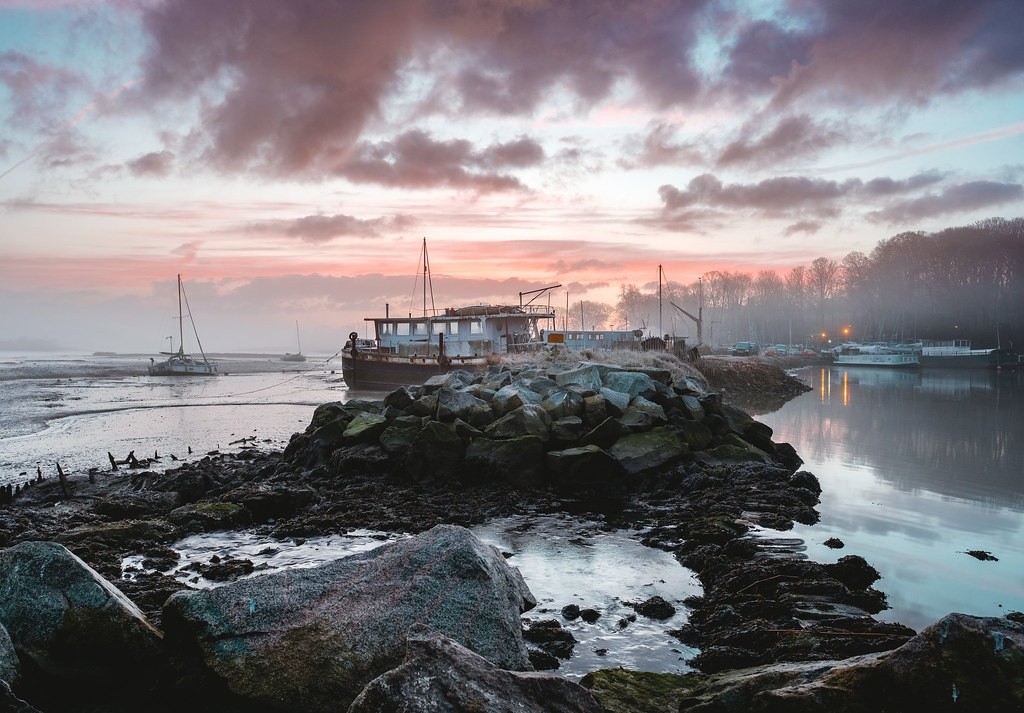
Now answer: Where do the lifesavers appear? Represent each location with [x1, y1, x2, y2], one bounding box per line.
[437, 355, 448, 365]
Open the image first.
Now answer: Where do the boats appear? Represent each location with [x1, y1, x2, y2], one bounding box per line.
[921, 338, 1024, 369]
[833, 341, 920, 366]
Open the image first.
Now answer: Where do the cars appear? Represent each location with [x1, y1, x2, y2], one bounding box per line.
[732, 340, 818, 358]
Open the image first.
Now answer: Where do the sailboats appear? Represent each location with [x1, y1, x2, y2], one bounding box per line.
[341, 236, 568, 390]
[149, 273, 219, 376]
[280, 319, 307, 362]
